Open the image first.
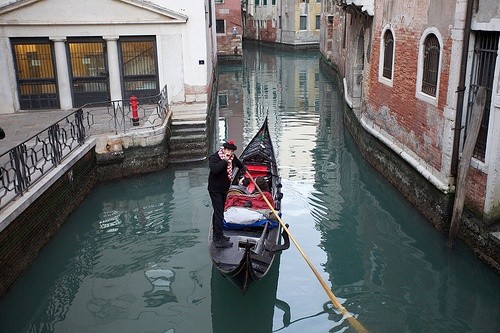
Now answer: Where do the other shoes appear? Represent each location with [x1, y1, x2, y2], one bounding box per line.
[222, 233, 230, 239]
[214, 235, 233, 247]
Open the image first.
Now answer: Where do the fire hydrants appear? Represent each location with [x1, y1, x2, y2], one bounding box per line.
[127, 95, 141, 127]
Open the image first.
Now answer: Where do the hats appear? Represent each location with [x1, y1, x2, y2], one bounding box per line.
[223, 141, 237, 149]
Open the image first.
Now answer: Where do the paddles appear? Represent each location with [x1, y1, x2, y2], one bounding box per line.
[244, 168, 370, 333]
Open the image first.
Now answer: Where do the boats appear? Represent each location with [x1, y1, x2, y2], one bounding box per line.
[207, 110, 291, 294]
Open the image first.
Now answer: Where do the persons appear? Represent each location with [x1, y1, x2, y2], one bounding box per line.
[209, 140, 249, 248]
